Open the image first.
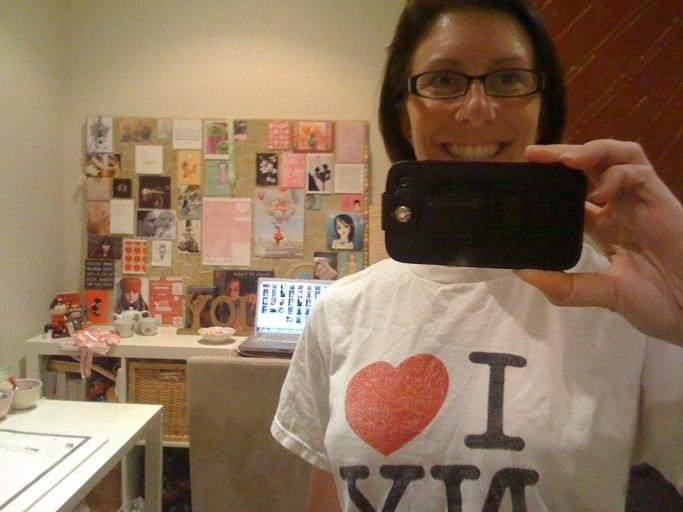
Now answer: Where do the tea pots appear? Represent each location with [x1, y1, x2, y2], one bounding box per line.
[114, 306, 151, 331]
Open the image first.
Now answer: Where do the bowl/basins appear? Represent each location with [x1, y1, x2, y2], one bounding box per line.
[199, 327, 236, 342]
[0, 379, 43, 419]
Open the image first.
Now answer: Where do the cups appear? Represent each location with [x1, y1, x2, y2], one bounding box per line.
[140, 318, 159, 335]
[116, 320, 137, 338]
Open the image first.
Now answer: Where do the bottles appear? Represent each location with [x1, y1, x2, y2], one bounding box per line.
[52, 297, 67, 328]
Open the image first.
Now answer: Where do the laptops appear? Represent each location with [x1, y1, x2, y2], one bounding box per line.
[238, 276, 338, 354]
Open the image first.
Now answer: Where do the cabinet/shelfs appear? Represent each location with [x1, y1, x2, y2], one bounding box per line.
[25, 326, 247, 512]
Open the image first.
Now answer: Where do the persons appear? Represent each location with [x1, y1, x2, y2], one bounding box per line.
[116, 278, 148, 317]
[137, 210, 175, 238]
[271, 0, 683, 512]
[331, 214, 355, 249]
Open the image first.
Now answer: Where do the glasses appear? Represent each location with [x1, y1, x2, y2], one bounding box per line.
[409, 70, 545, 97]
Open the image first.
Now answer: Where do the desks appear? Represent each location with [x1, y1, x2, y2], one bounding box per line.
[0, 397, 164, 512]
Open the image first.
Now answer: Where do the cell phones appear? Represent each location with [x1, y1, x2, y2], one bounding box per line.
[380, 158, 588, 272]
